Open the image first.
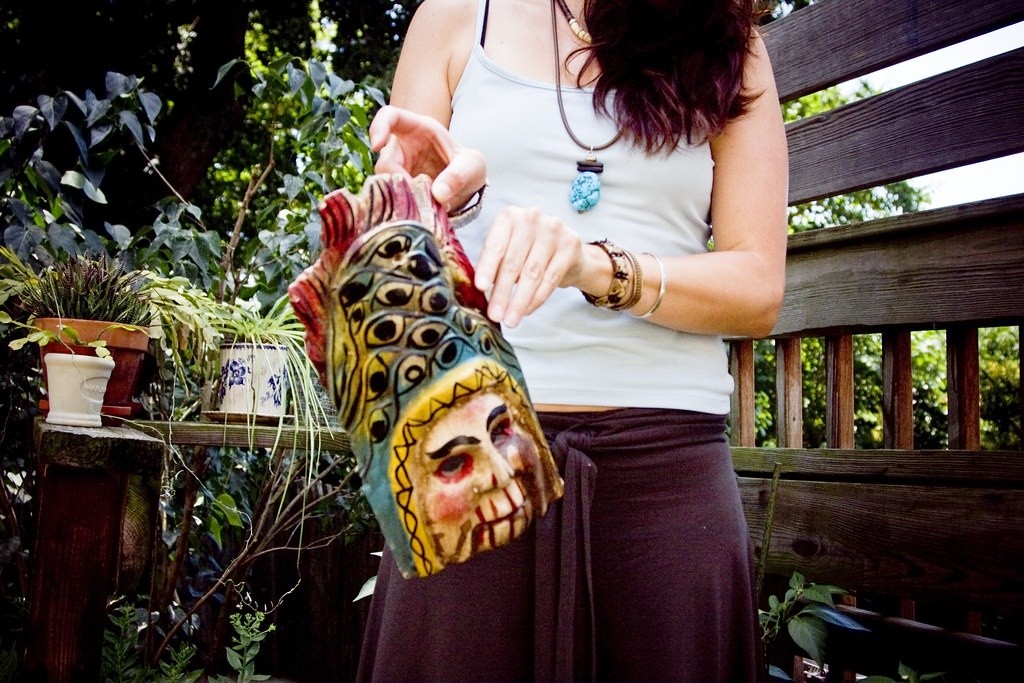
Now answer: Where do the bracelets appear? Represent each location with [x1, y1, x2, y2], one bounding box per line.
[446, 182, 487, 220]
[580, 237, 644, 312]
[628, 249, 670, 320]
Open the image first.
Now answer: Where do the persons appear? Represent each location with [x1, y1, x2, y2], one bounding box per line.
[354, 0, 789, 683]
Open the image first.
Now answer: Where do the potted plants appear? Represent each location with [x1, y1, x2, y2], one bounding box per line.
[200, 294, 335, 493]
[0, 308, 151, 427]
[0, 249, 168, 426]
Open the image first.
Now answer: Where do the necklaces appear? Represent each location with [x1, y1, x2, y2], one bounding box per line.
[551, 0, 629, 215]
